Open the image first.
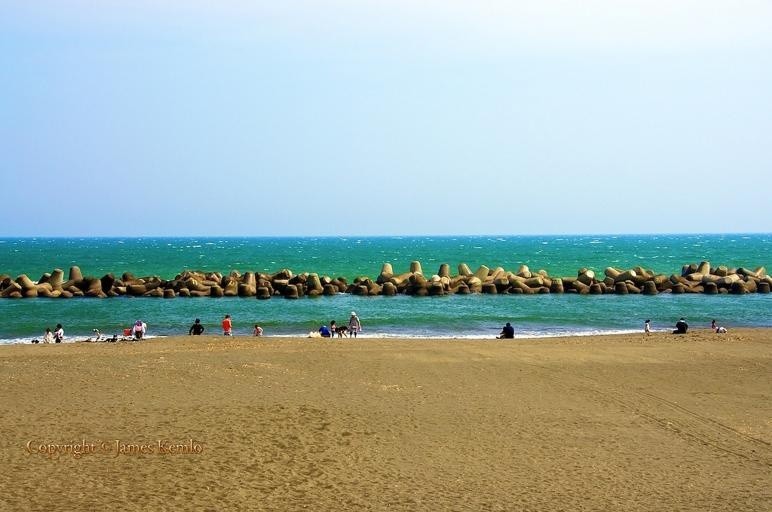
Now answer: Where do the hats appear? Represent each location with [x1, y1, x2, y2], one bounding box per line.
[350, 312, 357, 315]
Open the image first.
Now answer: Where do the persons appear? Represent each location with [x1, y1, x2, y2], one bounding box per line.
[645, 320, 652, 336]
[53, 324, 64, 343]
[330, 320, 336, 337]
[254, 325, 263, 336]
[496, 322, 514, 339]
[189, 319, 204, 335]
[335, 326, 348, 338]
[673, 318, 689, 333]
[319, 324, 330, 337]
[712, 320, 716, 331]
[715, 327, 727, 333]
[348, 311, 361, 338]
[42, 328, 54, 344]
[223, 315, 232, 336]
